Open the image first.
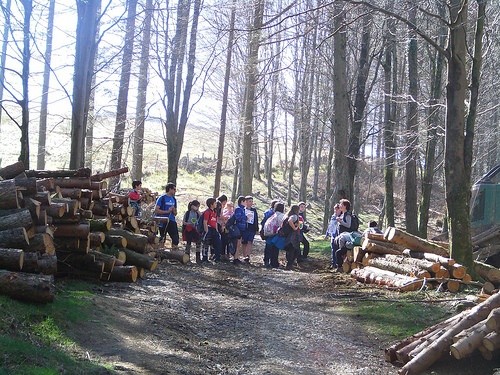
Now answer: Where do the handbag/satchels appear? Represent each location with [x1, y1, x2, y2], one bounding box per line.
[272, 234, 288, 249]
[277, 216, 295, 236]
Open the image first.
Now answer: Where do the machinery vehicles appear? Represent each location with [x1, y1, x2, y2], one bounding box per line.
[467, 163, 500, 270]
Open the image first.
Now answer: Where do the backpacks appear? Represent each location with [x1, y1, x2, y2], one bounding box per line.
[196, 212, 206, 238]
[344, 212, 359, 231]
[264, 213, 280, 236]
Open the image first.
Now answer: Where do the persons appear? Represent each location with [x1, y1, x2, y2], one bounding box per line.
[324, 202, 344, 271]
[362, 220, 383, 237]
[129, 181, 144, 216]
[202, 197, 223, 263]
[229, 195, 252, 264]
[329, 198, 363, 273]
[153, 182, 179, 252]
[281, 204, 301, 270]
[295, 201, 313, 261]
[263, 201, 288, 267]
[260, 200, 281, 266]
[241, 195, 259, 264]
[213, 194, 235, 258]
[182, 200, 204, 263]
